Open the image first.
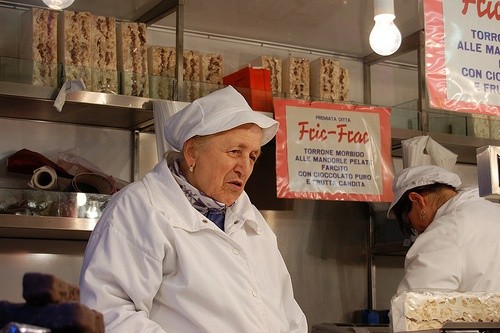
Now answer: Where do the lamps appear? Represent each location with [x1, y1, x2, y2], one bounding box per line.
[368, 0, 402, 56]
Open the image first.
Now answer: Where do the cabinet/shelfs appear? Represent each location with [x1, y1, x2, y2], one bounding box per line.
[0, 0, 500, 309]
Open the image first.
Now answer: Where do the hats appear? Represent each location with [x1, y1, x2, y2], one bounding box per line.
[163, 85, 279, 150]
[387, 165, 462, 219]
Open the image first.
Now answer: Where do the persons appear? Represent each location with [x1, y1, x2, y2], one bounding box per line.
[80, 85, 308, 333]
[387, 165, 500, 333]
